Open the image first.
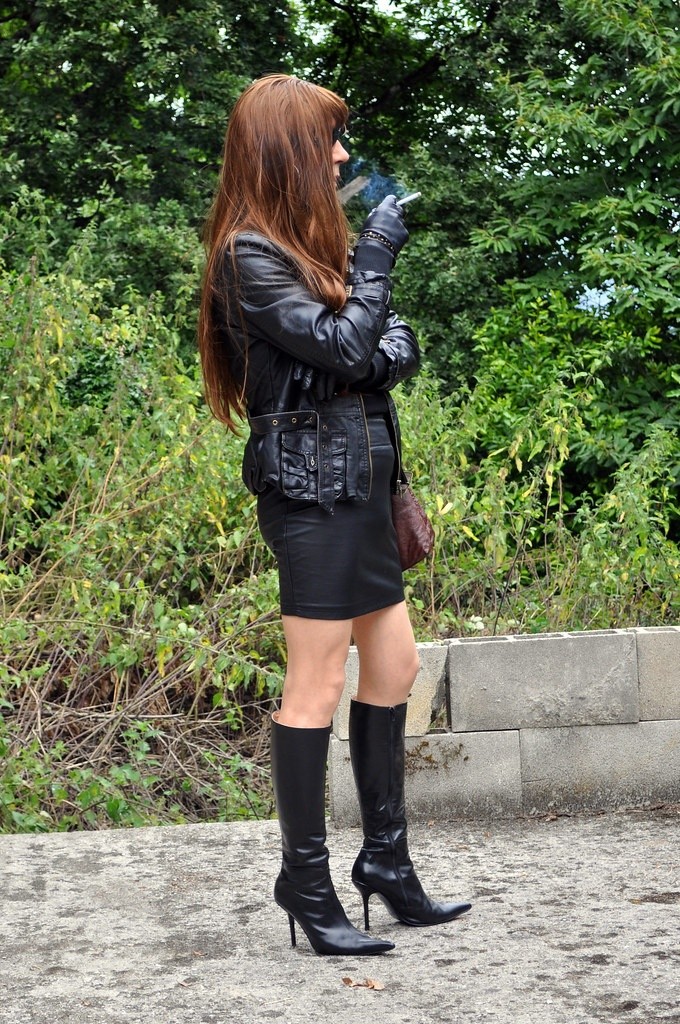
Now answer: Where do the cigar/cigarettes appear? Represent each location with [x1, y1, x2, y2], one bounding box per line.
[396, 191, 421, 205]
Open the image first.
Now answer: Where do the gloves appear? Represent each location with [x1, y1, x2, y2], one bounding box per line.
[360, 194, 410, 254]
[292, 359, 337, 403]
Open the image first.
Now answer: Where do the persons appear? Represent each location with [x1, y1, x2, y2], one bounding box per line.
[198, 73, 473, 954]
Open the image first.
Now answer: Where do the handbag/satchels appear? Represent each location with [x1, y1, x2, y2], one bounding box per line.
[391, 479, 436, 572]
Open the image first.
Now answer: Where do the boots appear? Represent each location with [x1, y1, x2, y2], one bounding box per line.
[349, 700, 472, 932]
[270, 710, 395, 955]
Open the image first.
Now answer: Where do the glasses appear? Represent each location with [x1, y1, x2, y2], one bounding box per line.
[332, 127, 346, 145]
